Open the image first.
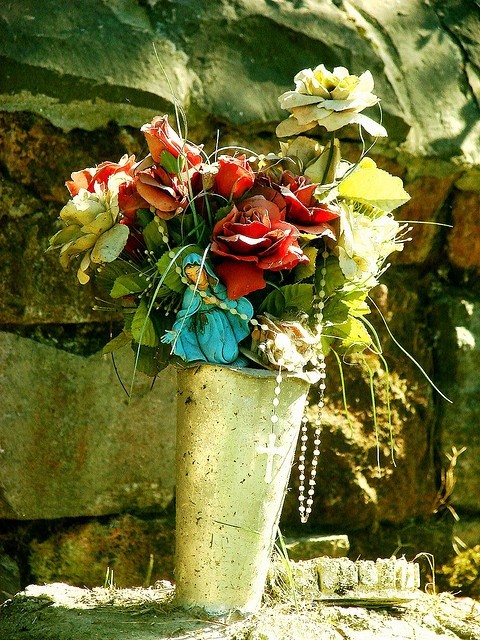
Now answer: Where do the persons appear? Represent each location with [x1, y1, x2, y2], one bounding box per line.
[159, 252, 255, 368]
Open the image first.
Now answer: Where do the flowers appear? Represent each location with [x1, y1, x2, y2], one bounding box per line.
[46, 63, 452, 464]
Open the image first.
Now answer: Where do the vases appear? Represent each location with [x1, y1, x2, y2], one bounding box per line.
[174, 361, 311, 616]
